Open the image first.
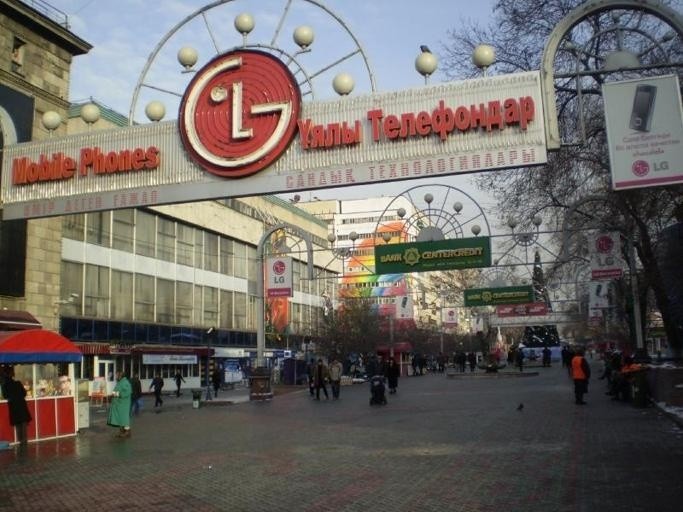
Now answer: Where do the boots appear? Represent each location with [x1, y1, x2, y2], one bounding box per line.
[115, 429, 131, 439]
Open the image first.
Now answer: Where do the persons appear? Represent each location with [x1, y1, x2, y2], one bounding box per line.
[569, 347, 590, 405]
[105, 368, 133, 438]
[312, 358, 328, 400]
[595, 346, 653, 402]
[305, 357, 317, 397]
[148, 371, 163, 408]
[384, 357, 400, 395]
[541, 345, 552, 367]
[172, 369, 185, 397]
[0, 369, 32, 447]
[327, 357, 343, 402]
[128, 372, 143, 416]
[561, 345, 593, 378]
[410, 346, 525, 378]
[211, 364, 224, 398]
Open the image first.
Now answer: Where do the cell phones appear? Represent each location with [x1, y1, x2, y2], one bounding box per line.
[629, 84, 657, 132]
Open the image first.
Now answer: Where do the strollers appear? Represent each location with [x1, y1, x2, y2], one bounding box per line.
[369, 375, 387, 405]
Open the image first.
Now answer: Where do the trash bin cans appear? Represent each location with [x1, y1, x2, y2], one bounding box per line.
[249, 376, 273, 401]
[623, 363, 682, 406]
